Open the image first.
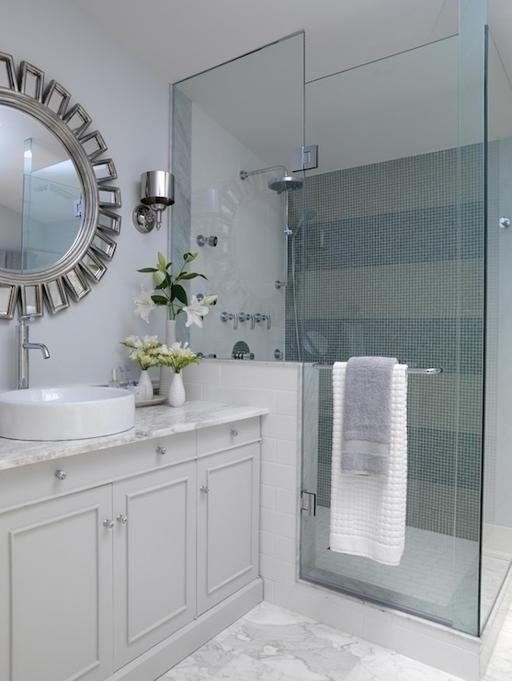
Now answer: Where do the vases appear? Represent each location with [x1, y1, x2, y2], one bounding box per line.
[166, 374, 190, 410]
[133, 371, 157, 408]
[161, 321, 181, 402]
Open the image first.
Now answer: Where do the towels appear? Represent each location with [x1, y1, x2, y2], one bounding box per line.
[341, 356, 398, 484]
[329, 361, 410, 566]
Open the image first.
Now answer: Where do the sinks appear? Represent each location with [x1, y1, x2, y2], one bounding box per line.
[0, 385, 136, 442]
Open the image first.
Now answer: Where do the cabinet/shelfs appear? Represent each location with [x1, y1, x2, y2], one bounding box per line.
[196, 418, 262, 649]
[0, 432, 197, 680]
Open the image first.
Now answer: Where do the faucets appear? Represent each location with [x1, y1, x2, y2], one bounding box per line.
[18, 315, 50, 390]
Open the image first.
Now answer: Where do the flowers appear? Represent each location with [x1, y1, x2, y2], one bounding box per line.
[119, 333, 163, 370]
[154, 342, 198, 374]
[137, 248, 223, 327]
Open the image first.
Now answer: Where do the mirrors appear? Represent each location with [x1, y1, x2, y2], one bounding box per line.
[0, 51, 122, 321]
